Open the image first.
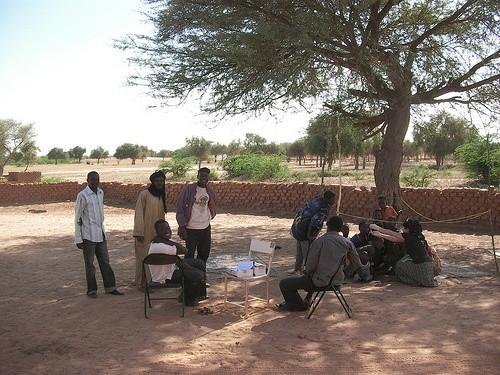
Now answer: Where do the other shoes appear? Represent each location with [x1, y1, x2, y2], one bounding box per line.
[88, 292, 97, 298]
[140, 287, 154, 293]
[107, 289, 124, 295]
[275, 301, 287, 310]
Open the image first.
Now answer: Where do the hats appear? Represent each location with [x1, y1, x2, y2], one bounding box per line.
[150, 170, 166, 182]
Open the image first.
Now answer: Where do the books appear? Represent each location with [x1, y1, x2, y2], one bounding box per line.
[225, 259, 266, 277]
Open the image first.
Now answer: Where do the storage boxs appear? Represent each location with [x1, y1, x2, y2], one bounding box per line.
[233, 260, 266, 277]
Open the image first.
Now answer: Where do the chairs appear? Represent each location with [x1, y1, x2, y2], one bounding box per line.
[141, 252, 186, 319]
[305, 253, 353, 320]
[223, 239, 276, 311]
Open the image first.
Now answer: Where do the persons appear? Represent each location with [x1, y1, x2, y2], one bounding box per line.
[73, 170, 124, 300]
[338, 195, 443, 289]
[132, 171, 168, 293]
[147, 219, 208, 307]
[288, 189, 337, 275]
[175, 167, 217, 288]
[274, 215, 361, 312]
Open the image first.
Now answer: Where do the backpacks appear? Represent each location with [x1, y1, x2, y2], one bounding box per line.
[291, 203, 319, 241]
